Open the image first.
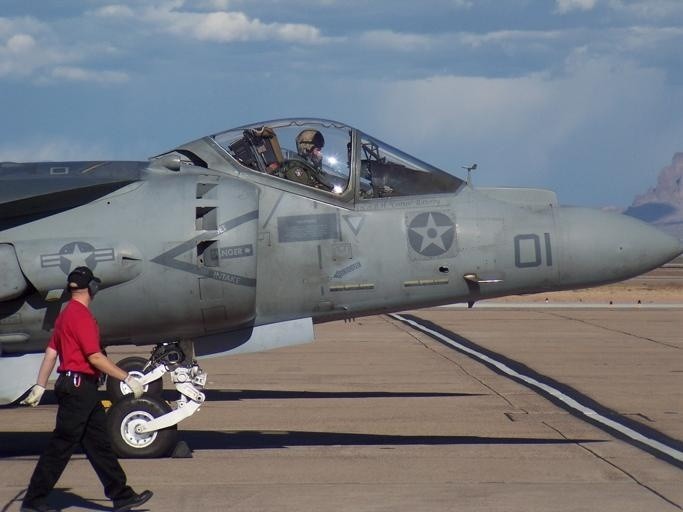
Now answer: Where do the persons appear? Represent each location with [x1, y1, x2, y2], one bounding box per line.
[20, 266, 153, 512]
[279, 128, 334, 192]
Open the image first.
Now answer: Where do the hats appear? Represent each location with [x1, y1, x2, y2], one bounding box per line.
[67, 267, 101, 289]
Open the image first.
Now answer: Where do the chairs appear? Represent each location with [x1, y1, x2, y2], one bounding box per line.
[246, 125, 283, 175]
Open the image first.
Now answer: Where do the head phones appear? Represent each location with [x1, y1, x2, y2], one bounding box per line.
[65, 269, 98, 297]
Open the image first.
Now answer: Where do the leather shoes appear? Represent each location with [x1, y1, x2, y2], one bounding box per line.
[113, 490, 153, 512]
[20, 503, 60, 512]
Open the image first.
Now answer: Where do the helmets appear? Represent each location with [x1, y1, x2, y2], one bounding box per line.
[295, 129, 325, 156]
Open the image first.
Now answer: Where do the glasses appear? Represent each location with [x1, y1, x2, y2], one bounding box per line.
[313, 146, 321, 150]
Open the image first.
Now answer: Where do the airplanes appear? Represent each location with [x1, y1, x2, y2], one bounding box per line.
[0, 113, 682, 462]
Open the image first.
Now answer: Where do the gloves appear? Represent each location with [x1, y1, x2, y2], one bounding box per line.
[20, 385, 46, 407]
[124, 374, 144, 399]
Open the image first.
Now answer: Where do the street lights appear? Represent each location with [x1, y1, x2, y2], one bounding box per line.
[458, 162, 478, 186]
